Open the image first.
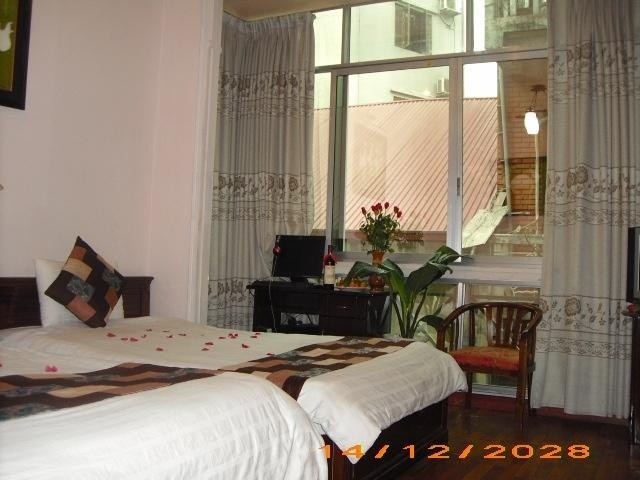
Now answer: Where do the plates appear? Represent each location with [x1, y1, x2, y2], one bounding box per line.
[338, 287, 367, 292]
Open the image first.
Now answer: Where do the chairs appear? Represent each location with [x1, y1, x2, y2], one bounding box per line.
[437, 301, 544, 434]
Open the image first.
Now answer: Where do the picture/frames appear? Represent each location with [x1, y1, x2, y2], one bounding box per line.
[0, 0, 33, 110]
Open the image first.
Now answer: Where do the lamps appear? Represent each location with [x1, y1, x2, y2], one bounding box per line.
[522, 82, 543, 135]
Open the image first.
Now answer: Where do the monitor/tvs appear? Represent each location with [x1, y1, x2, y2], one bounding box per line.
[271, 235, 326, 288]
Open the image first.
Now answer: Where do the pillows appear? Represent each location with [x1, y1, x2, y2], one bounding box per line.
[44, 235, 127, 329]
[34, 258, 125, 328]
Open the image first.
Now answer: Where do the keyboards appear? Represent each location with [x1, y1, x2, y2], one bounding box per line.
[252, 280, 308, 288]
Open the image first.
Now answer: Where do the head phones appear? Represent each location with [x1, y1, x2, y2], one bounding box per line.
[273, 235, 282, 255]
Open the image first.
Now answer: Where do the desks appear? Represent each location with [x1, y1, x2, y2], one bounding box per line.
[246, 280, 392, 335]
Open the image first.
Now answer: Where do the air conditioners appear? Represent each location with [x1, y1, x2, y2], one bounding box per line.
[437, 78, 449, 95]
[439, 0, 462, 16]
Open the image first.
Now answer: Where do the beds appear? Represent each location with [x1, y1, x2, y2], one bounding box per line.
[2, 340, 328, 478]
[2, 276, 470, 479]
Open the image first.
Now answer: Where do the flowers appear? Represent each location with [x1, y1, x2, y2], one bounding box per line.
[359, 202, 402, 250]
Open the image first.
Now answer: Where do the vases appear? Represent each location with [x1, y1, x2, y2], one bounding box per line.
[369, 252, 384, 292]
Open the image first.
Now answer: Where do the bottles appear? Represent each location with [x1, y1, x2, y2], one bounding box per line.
[323, 244, 336, 293]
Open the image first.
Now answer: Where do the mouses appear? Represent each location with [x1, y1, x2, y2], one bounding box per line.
[313, 285, 324, 288]
